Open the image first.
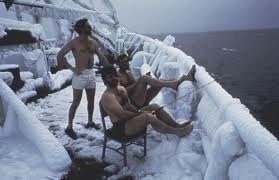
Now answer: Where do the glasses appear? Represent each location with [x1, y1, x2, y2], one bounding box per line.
[120, 59, 130, 64]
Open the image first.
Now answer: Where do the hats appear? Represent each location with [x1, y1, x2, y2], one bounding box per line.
[101, 65, 117, 79]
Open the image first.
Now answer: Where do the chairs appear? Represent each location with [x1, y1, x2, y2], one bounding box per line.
[99, 101, 147, 166]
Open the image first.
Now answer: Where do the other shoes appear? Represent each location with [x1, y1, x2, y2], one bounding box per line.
[65, 126, 78, 141]
[87, 122, 102, 130]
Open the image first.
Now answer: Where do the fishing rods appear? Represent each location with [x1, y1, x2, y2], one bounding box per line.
[152, 77, 220, 114]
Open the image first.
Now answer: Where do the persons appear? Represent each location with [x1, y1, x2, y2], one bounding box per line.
[117, 54, 196, 109]
[58, 18, 111, 139]
[102, 65, 193, 140]
